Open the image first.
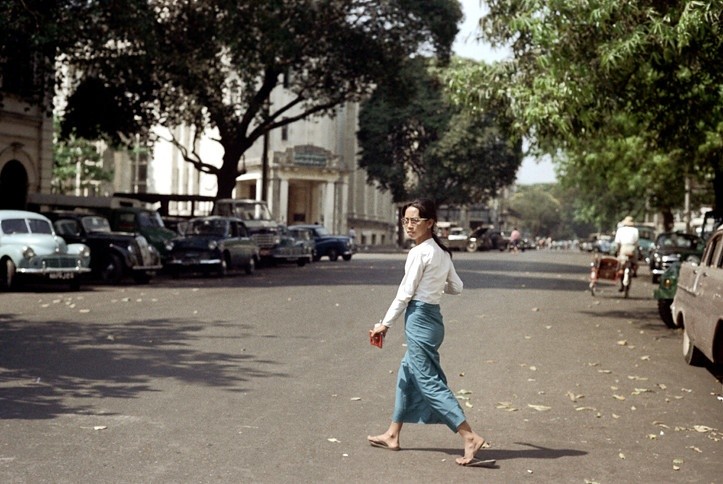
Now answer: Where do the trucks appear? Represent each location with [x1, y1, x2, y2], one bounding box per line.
[27, 193, 179, 263]
[112, 192, 284, 270]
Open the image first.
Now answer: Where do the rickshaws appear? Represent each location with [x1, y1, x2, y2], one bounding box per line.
[588, 252, 644, 299]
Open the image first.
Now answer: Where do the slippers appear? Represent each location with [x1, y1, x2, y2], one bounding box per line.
[456, 457, 496, 466]
[368, 435, 401, 451]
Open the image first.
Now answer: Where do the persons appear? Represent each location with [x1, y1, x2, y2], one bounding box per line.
[367, 199, 497, 470]
[349, 227, 357, 244]
[614, 216, 639, 292]
[436, 222, 591, 254]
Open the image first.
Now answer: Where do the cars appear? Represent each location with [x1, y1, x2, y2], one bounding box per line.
[0, 210, 91, 292]
[647, 232, 706, 284]
[36, 210, 164, 285]
[653, 208, 723, 329]
[431, 218, 660, 261]
[288, 224, 355, 262]
[671, 224, 723, 366]
[163, 216, 261, 277]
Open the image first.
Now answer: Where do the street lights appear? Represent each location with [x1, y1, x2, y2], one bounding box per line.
[259, 93, 271, 204]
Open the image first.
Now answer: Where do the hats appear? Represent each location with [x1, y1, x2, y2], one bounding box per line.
[621, 216, 636, 226]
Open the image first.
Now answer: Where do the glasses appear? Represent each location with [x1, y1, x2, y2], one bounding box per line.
[401, 217, 429, 225]
